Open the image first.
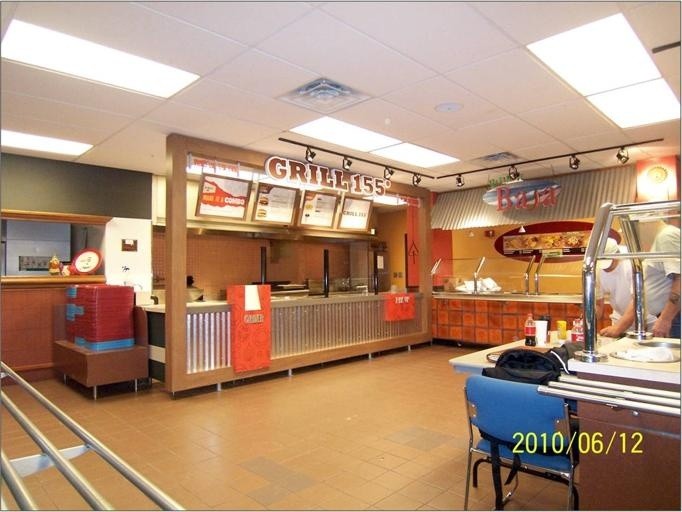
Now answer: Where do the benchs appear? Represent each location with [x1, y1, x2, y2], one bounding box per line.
[51, 306, 149, 400]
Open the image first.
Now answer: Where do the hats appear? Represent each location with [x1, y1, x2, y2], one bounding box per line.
[598, 238, 619, 270]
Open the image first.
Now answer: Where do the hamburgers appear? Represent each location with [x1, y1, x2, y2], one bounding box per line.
[260, 197, 268, 206]
[258, 209, 266, 218]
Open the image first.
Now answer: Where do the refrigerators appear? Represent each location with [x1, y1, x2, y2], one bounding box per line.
[349, 241, 390, 291]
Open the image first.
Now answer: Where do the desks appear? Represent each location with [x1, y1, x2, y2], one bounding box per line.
[446, 329, 627, 382]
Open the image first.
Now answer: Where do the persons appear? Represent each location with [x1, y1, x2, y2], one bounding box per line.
[595, 237, 636, 337]
[600, 219, 682, 338]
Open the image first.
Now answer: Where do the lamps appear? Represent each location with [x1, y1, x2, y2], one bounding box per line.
[275, 132, 436, 191]
[433, 128, 666, 187]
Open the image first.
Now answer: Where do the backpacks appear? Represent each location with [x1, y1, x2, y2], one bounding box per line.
[479, 347, 569, 484]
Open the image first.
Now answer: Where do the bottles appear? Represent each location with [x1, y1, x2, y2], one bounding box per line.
[556, 320, 566, 338]
[524, 313, 536, 346]
[49, 252, 62, 275]
[539, 315, 550, 342]
[570, 319, 583, 341]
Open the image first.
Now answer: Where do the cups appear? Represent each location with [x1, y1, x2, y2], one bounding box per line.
[534, 320, 547, 346]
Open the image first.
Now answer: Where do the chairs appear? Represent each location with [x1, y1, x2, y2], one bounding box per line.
[461, 372, 580, 511]
